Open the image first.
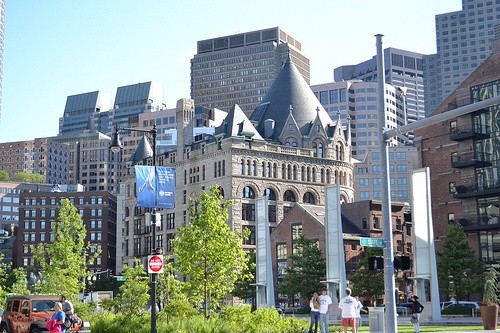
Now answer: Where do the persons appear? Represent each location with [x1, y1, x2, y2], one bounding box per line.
[408, 296, 424, 333]
[308, 292, 319, 333]
[51, 302, 66, 333]
[339, 288, 358, 333]
[59, 295, 74, 333]
[354, 296, 363, 331]
[318, 288, 332, 333]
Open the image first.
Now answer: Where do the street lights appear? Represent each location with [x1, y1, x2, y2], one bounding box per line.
[109, 122, 159, 333]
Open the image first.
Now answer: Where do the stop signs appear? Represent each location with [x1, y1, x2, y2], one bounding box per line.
[147, 255, 164, 273]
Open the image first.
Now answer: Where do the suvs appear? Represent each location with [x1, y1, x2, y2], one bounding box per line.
[0, 295, 84, 333]
[446, 301, 481, 310]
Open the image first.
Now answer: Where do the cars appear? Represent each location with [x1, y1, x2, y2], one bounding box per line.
[441, 302, 449, 310]
[275, 306, 298, 314]
